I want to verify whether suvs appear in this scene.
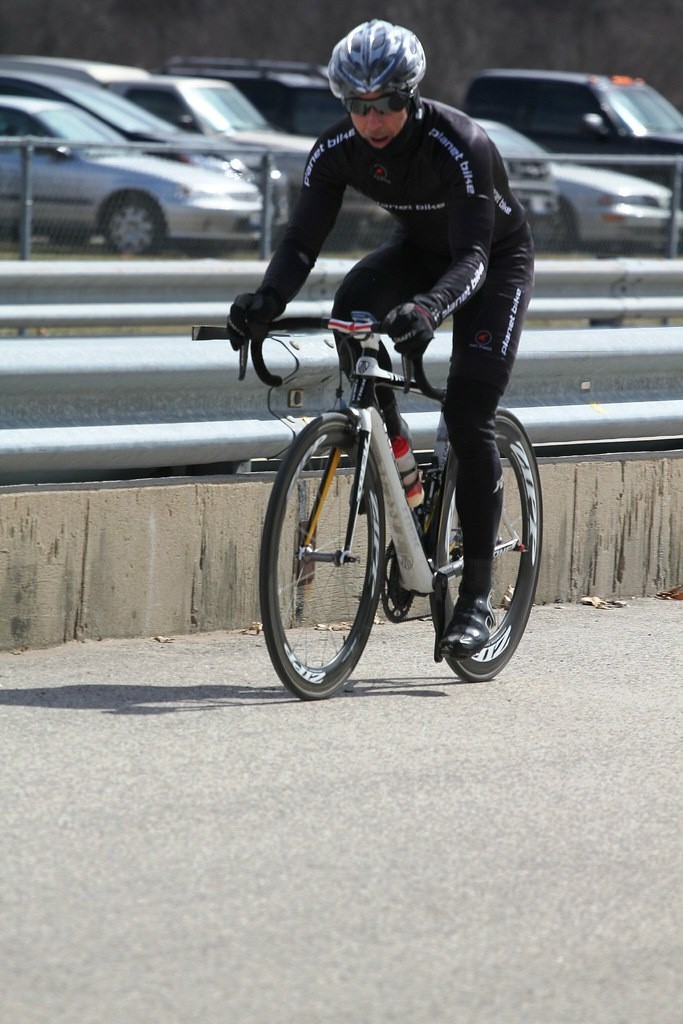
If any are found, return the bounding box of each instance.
[168,59,558,244]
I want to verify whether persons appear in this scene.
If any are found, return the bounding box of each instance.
[226,20,535,657]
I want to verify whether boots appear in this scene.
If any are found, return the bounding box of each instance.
[349,399,413,514]
[440,555,496,660]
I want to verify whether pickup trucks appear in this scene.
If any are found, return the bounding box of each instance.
[0,51,381,244]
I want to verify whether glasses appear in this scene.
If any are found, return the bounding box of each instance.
[345,93,407,116]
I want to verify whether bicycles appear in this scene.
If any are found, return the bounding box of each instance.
[192,312,544,699]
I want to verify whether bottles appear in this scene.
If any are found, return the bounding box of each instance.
[391,437,424,507]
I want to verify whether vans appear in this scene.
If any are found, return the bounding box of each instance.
[464,68,682,196]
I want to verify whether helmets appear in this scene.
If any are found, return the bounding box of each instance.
[328,19,426,99]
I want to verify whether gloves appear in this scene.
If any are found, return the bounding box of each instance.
[382,301,433,359]
[227,287,286,351]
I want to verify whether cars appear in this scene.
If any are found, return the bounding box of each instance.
[2,65,293,231]
[478,118,683,257]
[0,94,263,256]
